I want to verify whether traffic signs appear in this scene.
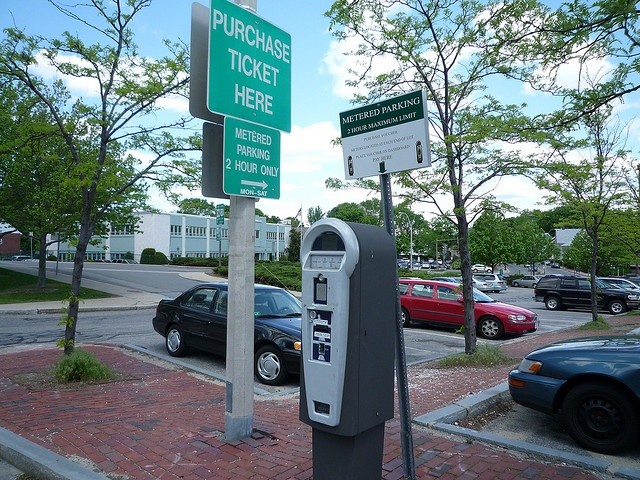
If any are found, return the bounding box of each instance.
[222,116,281,200]
[208,0,291,133]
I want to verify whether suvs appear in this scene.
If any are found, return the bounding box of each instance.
[535,275,640,314]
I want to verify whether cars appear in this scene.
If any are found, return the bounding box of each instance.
[551,262,560,268]
[398,278,539,338]
[545,260,551,266]
[512,275,543,289]
[454,277,490,290]
[529,265,537,271]
[510,326,640,455]
[470,263,493,273]
[396,257,452,271]
[430,277,457,283]
[524,264,533,267]
[597,278,640,292]
[151,282,302,385]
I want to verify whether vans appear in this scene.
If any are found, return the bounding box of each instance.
[471,273,508,293]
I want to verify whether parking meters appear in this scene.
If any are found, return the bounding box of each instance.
[297,217,395,480]
